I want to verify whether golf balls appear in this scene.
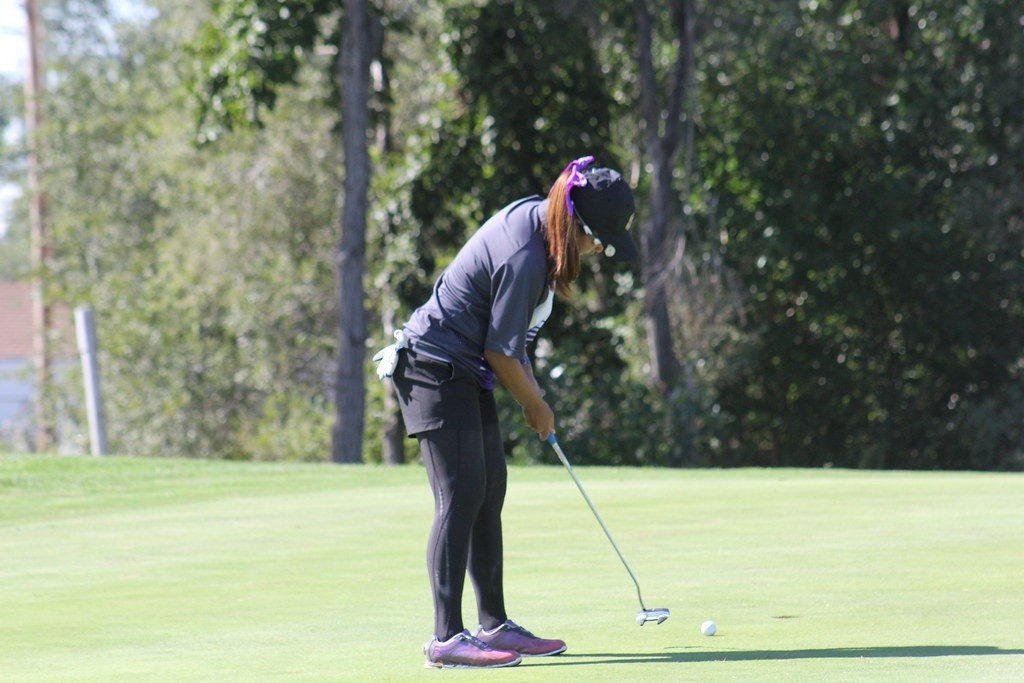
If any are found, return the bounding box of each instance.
[700,621,716,636]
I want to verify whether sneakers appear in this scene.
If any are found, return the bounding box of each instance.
[425,629,522,669]
[471,619,567,656]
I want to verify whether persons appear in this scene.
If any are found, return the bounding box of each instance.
[393,154,634,666]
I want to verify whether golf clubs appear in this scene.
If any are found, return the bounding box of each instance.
[545,432,670,626]
